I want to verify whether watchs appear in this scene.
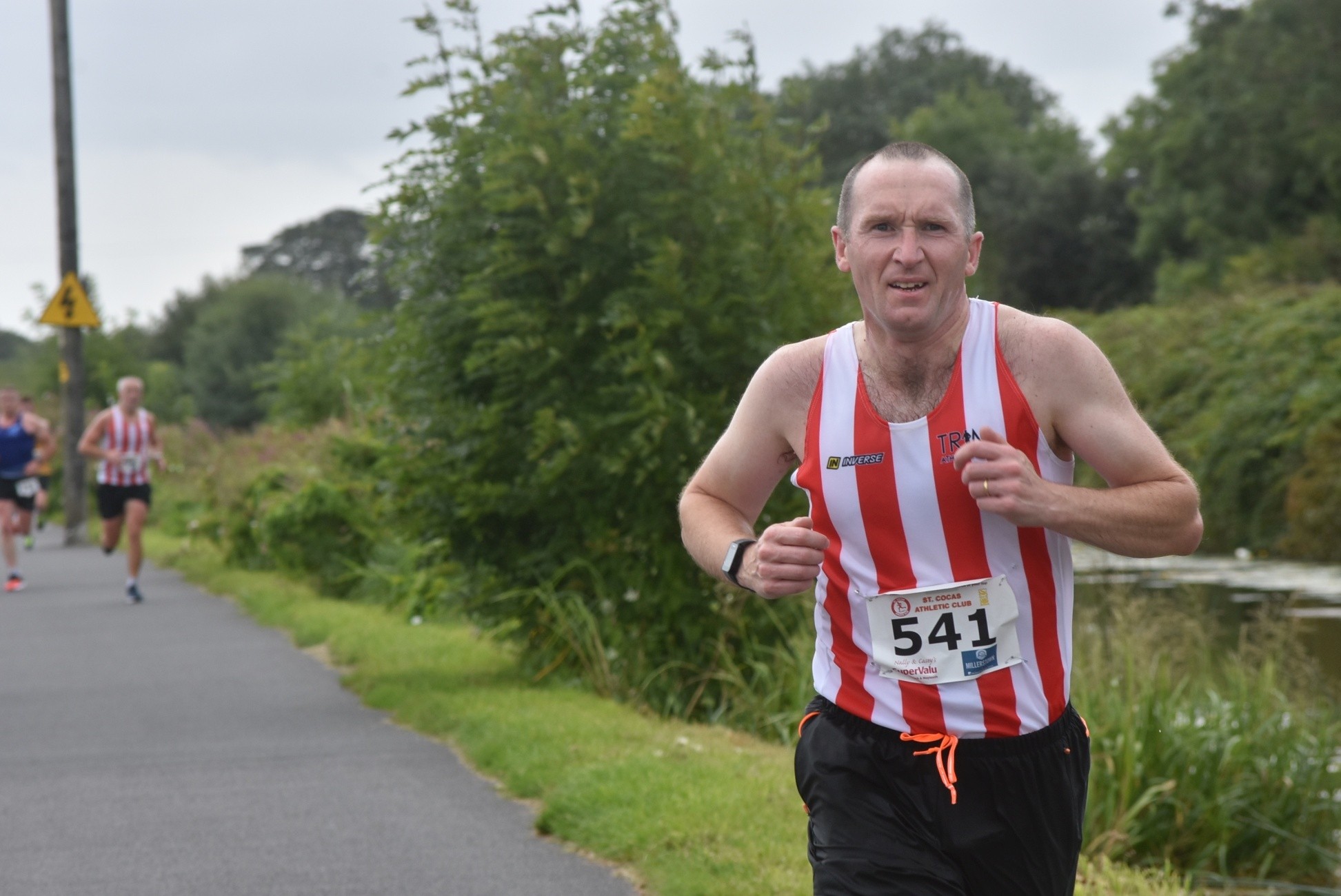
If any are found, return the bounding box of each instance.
[721,538,757,593]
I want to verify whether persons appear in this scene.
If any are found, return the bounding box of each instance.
[0,389,58,590]
[76,376,168,604]
[678,141,1204,896]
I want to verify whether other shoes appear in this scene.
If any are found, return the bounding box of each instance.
[4,574,26,592]
[102,549,114,555]
[22,534,33,551]
[123,586,142,605]
[34,510,47,530]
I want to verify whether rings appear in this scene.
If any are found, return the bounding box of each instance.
[983,479,991,498]
